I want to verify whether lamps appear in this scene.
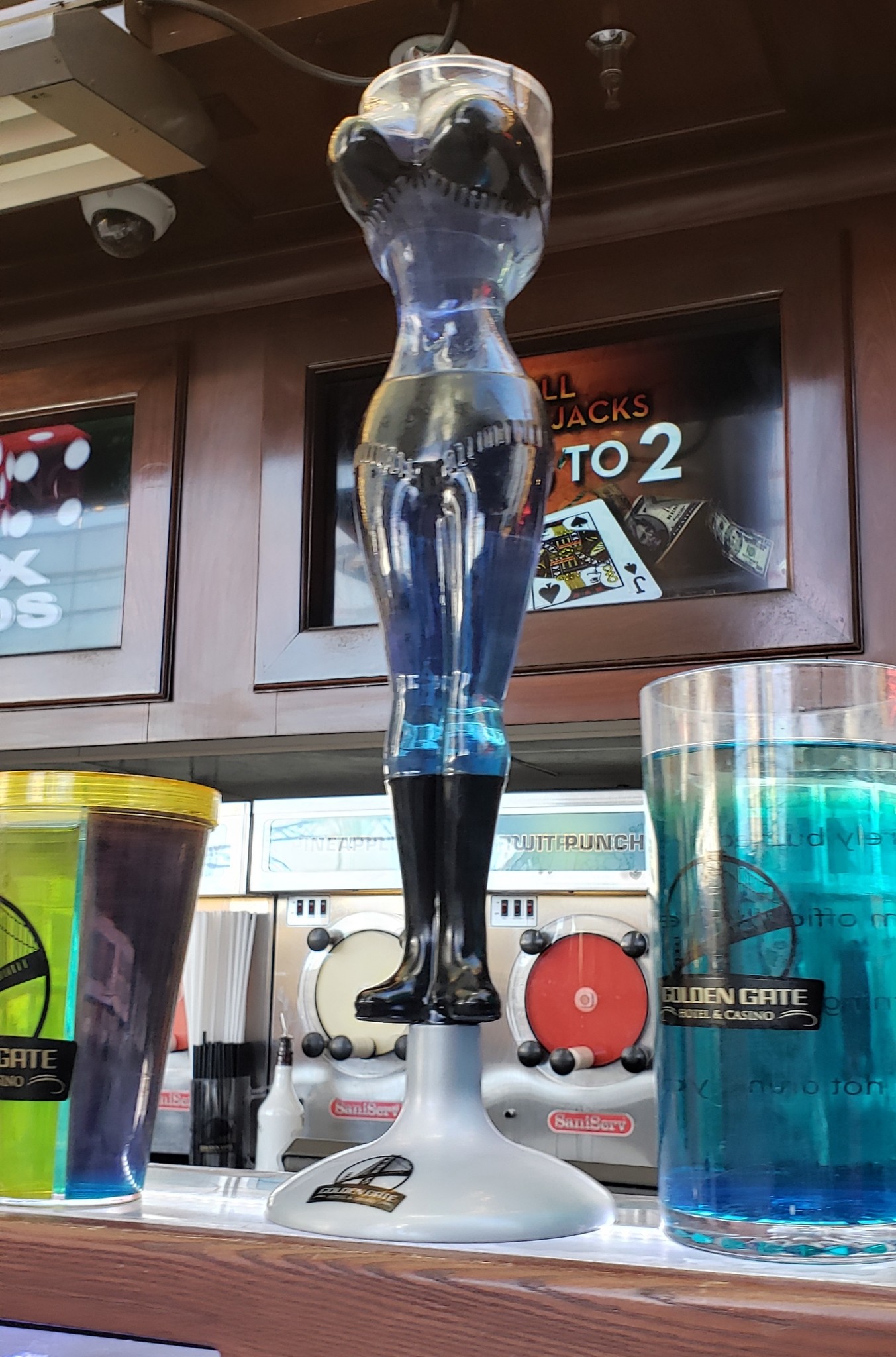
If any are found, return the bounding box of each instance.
[0,5,213,217]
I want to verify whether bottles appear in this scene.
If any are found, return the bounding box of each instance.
[255,1012,305,1172]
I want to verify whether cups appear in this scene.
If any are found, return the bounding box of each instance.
[0,770,221,1207]
[636,659,896,1266]
[187,1076,250,1168]
